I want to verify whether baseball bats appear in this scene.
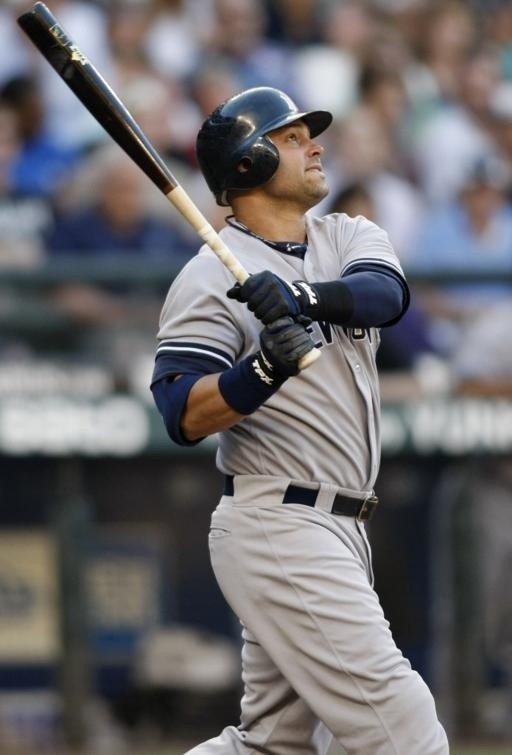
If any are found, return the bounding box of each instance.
[17,0,321,370]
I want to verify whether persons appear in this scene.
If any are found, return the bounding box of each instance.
[149,85,450,754]
[0,0,512,403]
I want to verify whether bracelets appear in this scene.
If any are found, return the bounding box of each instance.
[217,348,291,417]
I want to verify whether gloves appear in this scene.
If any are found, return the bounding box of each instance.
[227,268,323,325]
[239,317,311,390]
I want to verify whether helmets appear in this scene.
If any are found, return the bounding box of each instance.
[196,88,332,205]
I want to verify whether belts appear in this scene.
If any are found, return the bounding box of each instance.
[221,477,378,520]
